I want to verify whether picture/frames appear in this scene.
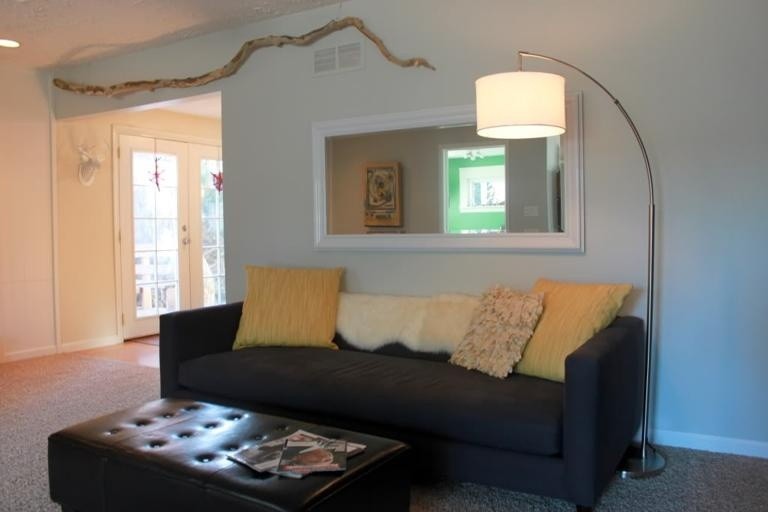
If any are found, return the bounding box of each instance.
[360,162,404,227]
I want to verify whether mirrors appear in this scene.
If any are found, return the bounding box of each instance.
[311,91,585,256]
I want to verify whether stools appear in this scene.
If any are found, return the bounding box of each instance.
[48,396,413,512]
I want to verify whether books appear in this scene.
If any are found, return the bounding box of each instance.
[226,429,368,480]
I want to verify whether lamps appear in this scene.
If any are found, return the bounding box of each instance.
[474,51,665,479]
[77,143,101,186]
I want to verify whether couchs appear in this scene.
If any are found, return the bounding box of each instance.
[160,264,646,512]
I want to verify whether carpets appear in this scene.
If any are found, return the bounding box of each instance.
[1,351,768,511]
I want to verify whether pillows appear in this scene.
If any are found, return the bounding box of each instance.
[336,291,481,355]
[515,278,632,384]
[233,264,345,351]
[448,284,544,380]
